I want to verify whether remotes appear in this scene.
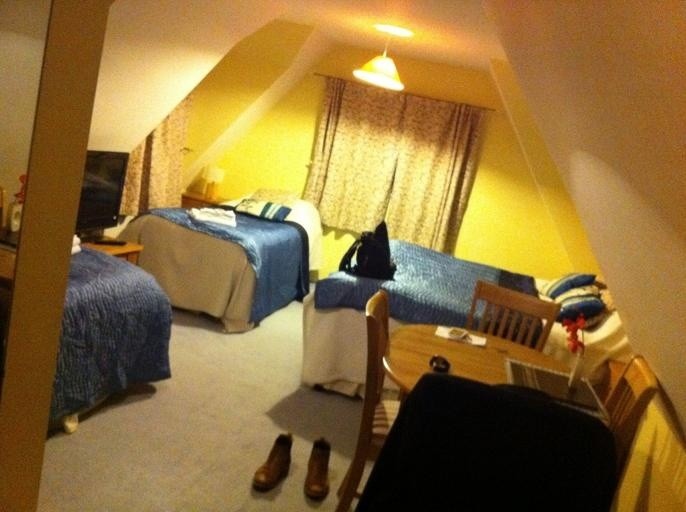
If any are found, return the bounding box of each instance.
[94,240,126,245]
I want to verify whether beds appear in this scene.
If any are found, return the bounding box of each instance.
[301,237,632,391]
[0,231,172,434]
[130,204,323,333]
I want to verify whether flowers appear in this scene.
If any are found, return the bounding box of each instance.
[562,313,586,355]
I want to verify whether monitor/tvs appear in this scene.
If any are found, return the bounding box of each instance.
[74,150,129,243]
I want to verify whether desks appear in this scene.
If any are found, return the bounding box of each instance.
[382,323,613,430]
[78,235,145,267]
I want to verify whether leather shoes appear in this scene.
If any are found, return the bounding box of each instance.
[252,434,292,492]
[303,439,330,501]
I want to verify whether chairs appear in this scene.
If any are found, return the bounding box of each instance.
[602,352,660,460]
[351,373,618,511]
[465,279,562,352]
[334,289,415,512]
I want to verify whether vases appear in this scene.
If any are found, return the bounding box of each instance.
[568,354,587,389]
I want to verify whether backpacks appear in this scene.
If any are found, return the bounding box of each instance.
[339,219,397,281]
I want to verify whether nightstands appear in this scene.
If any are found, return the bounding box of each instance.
[181,191,228,208]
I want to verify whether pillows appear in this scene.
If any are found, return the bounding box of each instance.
[537,271,606,330]
[235,199,290,221]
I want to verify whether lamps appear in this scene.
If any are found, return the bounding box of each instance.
[352,38,405,93]
